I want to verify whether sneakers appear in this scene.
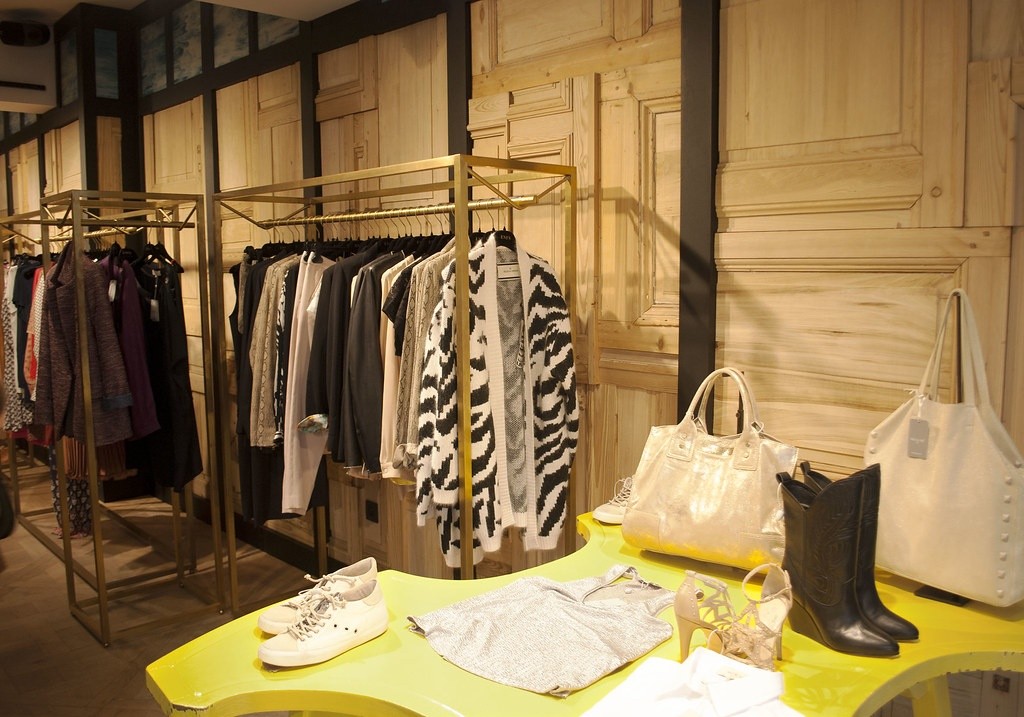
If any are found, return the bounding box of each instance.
[594,475,636,525]
[259,579,387,667]
[258,557,377,635]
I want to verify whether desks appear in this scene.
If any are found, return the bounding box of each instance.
[136,503,1024,717]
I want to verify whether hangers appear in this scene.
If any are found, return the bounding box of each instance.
[244,198,517,255]
[3,229,185,274]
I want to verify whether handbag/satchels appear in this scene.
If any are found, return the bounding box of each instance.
[864,288,1024,608]
[621,366,799,574]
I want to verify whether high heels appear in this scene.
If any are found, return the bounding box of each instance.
[727,562,793,666]
[674,570,750,663]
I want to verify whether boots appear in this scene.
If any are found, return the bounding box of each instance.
[800,461,918,643]
[776,472,900,657]
